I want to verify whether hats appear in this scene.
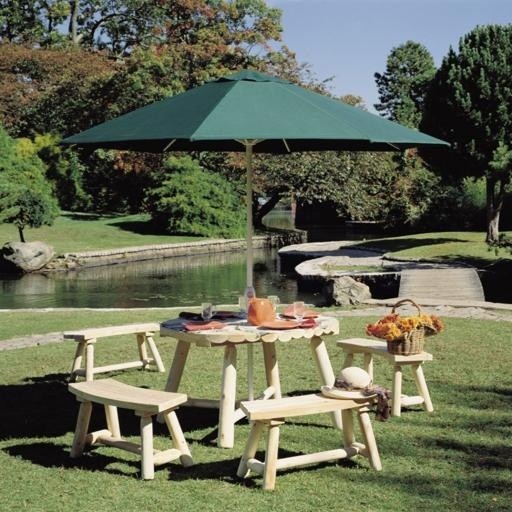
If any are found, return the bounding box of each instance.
[320,366,379,399]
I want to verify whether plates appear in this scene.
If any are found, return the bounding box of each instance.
[261,320,301,329]
[283,311,321,318]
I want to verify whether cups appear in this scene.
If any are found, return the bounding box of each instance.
[238,295,248,313]
[201,302,217,321]
[292,300,305,321]
[267,295,280,312]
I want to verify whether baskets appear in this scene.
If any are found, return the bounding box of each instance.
[386,298,424,355]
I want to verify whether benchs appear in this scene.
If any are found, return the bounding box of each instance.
[337,336,436,416]
[237,390,383,490]
[67,378,193,481]
[63,321,165,381]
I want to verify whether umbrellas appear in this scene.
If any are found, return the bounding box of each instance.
[54,66,455,401]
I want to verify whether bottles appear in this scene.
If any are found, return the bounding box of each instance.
[246,286,254,303]
